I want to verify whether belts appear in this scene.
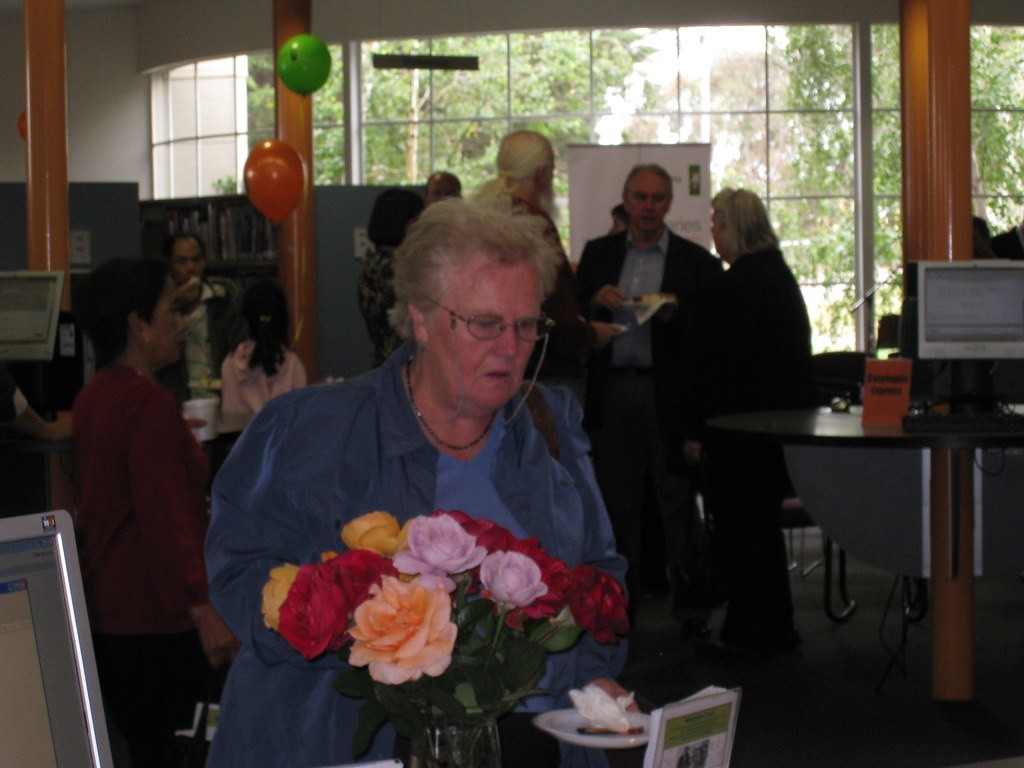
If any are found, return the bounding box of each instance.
[608,365,652,377]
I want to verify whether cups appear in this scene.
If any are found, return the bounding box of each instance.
[183,398,220,442]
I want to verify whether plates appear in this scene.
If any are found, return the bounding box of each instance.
[534,709,652,749]
[622,299,673,310]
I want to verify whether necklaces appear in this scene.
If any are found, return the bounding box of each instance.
[406,357,498,450]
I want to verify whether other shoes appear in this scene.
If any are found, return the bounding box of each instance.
[696,630,801,664]
[682,618,712,640]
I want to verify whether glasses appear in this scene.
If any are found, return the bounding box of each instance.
[421,293,556,341]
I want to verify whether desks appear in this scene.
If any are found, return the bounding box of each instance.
[708,403,1024,701]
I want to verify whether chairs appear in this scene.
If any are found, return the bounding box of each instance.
[772,352,873,623]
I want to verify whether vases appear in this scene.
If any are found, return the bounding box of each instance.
[405,715,500,768]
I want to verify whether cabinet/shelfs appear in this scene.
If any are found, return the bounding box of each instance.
[151,185,428,391]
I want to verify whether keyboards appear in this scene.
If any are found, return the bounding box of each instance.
[902,411,1024,435]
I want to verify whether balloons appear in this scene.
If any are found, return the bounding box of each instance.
[244,141,304,223]
[277,34,331,95]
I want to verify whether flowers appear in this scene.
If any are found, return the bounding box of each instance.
[255,506,628,724]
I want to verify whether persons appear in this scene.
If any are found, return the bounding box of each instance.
[71,256,239,768]
[573,165,722,634]
[0,367,75,511]
[155,233,249,390]
[682,190,812,667]
[973,215,1024,260]
[203,197,639,768]
[472,130,624,382]
[359,171,463,373]
[208,282,308,499]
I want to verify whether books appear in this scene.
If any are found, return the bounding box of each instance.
[169,204,274,260]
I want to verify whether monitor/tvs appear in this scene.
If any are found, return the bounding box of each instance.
[1,269,64,362]
[0,510,116,768]
[902,261,1024,411]
